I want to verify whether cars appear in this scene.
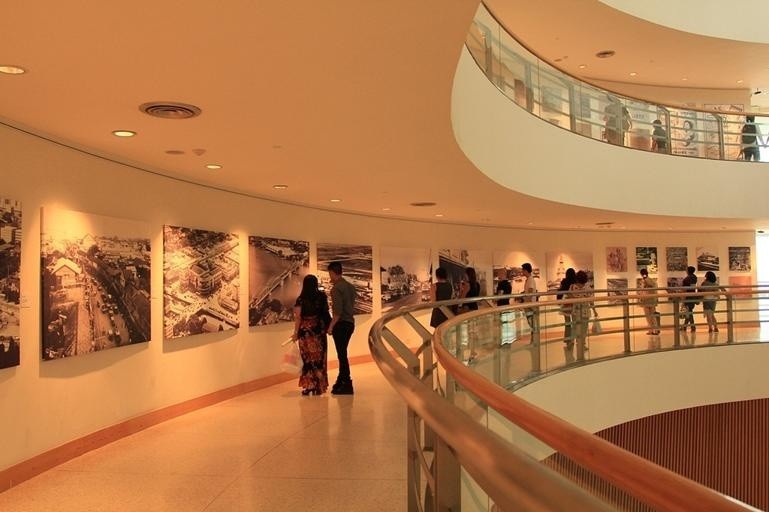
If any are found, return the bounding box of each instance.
[382,282,431,302]
[91,277,120,342]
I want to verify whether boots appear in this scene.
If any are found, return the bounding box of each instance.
[330,377,354,395]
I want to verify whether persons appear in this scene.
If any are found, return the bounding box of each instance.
[697,271,728,333]
[603,92,633,146]
[679,266,699,330]
[638,269,660,335]
[427,261,598,353]
[288,274,332,397]
[739,115,768,161]
[652,119,668,153]
[327,262,356,395]
[682,120,695,147]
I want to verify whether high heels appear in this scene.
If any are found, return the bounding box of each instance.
[302,388,320,394]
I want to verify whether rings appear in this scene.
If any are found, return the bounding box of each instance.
[329,331,332,334]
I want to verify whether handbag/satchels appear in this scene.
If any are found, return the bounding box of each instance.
[559,292,572,316]
